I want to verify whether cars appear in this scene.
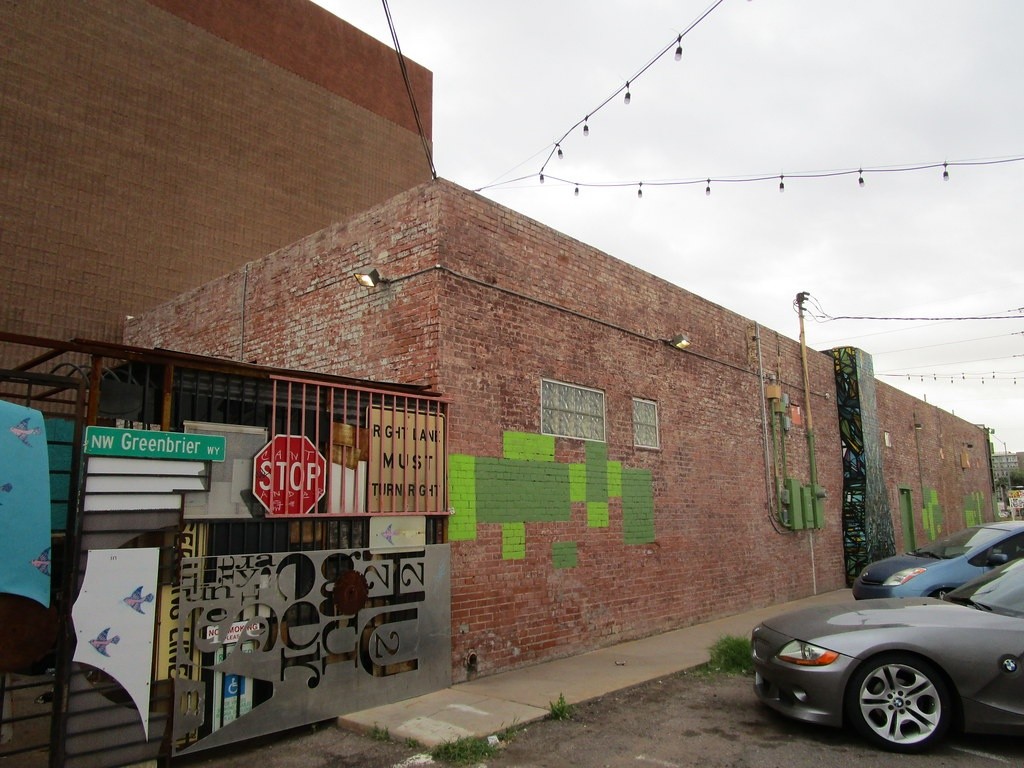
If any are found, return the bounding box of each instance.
[748,555,1024,752]
[852,518,1024,603]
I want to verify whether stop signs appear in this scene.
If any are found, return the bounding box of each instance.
[249,437,325,516]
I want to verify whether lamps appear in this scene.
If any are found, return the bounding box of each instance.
[667,333,691,349]
[963,442,973,448]
[354,267,388,288]
[913,424,922,429]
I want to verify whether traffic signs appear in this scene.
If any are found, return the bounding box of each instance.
[86,425,226,465]
